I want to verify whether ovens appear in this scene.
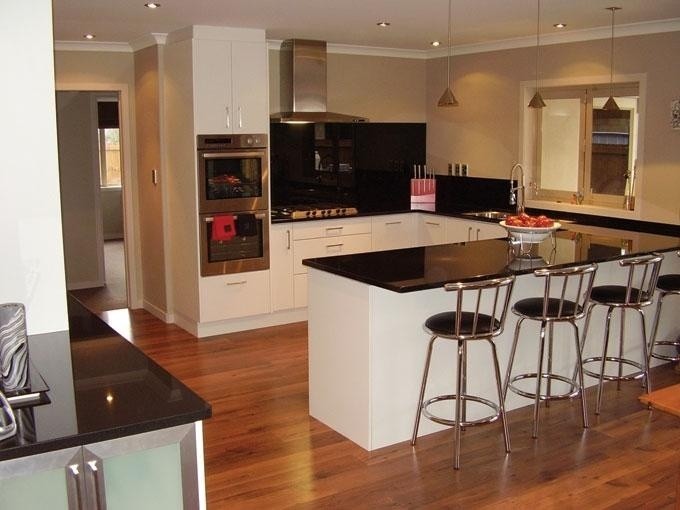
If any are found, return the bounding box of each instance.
[198,134,269,277]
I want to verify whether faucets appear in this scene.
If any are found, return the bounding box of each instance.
[509,163,524,213]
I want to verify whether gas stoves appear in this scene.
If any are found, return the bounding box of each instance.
[272,197,357,219]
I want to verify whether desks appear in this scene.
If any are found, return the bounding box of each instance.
[637,382,680,441]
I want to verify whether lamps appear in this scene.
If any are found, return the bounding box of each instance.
[528,0,547,108]
[437,0,459,108]
[602,7,621,114]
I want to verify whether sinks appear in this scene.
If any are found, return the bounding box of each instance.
[463,210,509,221]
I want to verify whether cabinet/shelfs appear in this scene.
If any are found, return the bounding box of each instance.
[269,219,294,327]
[295,216,372,324]
[169,269,272,339]
[0,408,209,510]
[165,38,270,134]
[373,211,419,252]
[447,216,508,244]
[419,213,447,247]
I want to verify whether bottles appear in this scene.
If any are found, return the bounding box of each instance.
[623,169,631,209]
[631,166,636,210]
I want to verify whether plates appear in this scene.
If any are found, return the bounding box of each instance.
[500,221,561,242]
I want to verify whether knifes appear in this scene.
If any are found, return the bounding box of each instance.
[413,164,435,178]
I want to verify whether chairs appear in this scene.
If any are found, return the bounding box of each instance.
[411,275,511,470]
[648,249,680,368]
[570,251,664,415]
[502,263,599,438]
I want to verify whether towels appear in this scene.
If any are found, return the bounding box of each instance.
[236,215,257,237]
[212,215,236,240]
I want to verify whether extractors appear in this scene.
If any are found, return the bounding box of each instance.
[270,38,369,125]
